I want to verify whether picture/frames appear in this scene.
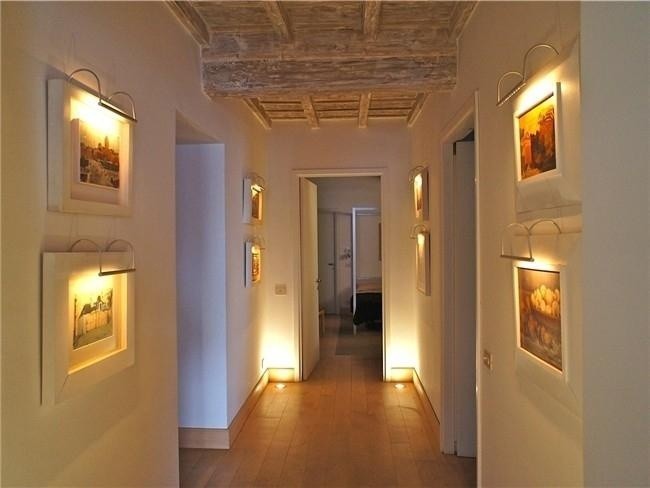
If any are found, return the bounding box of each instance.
[512,260,569,380]
[513,82,562,185]
[413,167,432,297]
[46,78,134,218]
[39,252,135,405]
[242,177,263,288]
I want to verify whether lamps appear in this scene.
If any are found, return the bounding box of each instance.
[499,217,563,264]
[69,239,138,278]
[67,68,139,125]
[494,43,561,108]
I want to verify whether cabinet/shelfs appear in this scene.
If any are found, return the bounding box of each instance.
[317,211,353,315]
